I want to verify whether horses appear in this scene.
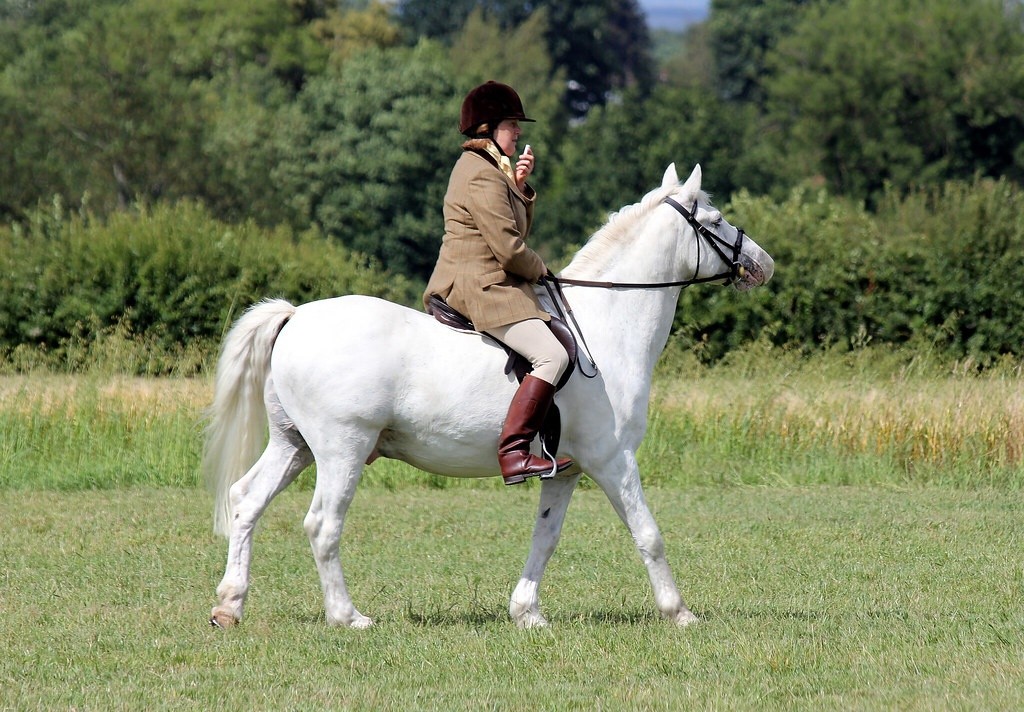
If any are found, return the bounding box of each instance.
[198,161,776,635]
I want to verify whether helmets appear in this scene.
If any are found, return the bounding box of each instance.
[460,81,536,135]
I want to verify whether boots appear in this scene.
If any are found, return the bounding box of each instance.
[497,373,574,485]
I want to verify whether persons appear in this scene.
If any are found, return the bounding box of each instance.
[423,80,573,485]
